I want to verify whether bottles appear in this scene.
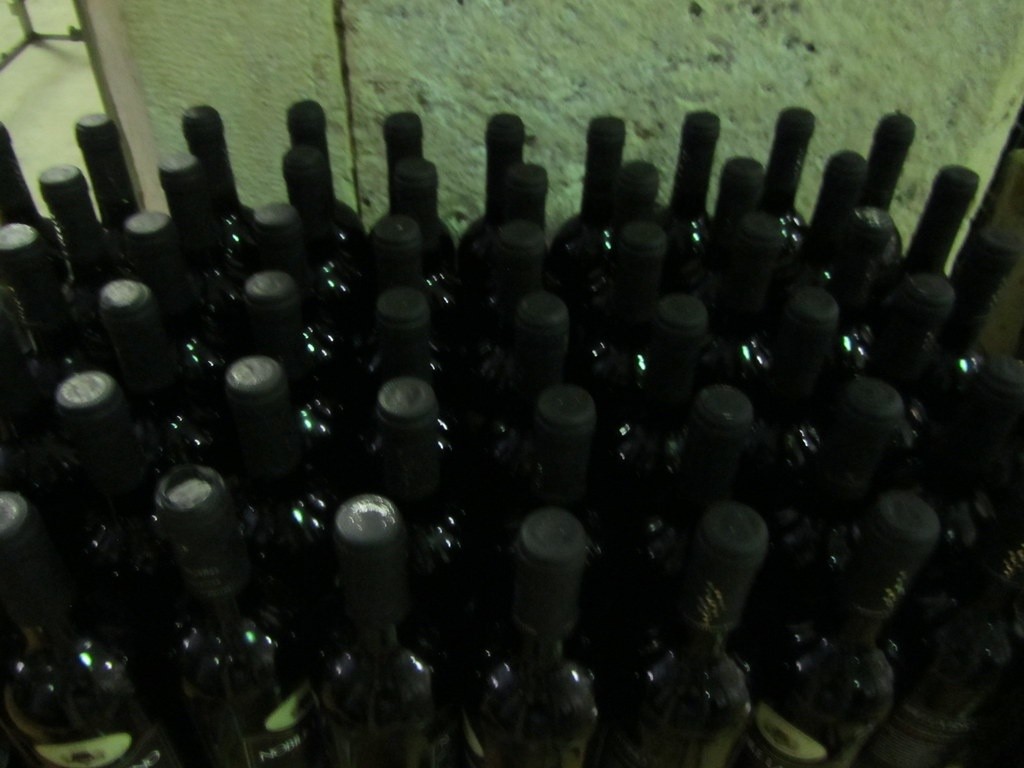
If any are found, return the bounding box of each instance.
[0,88,1024,768]
